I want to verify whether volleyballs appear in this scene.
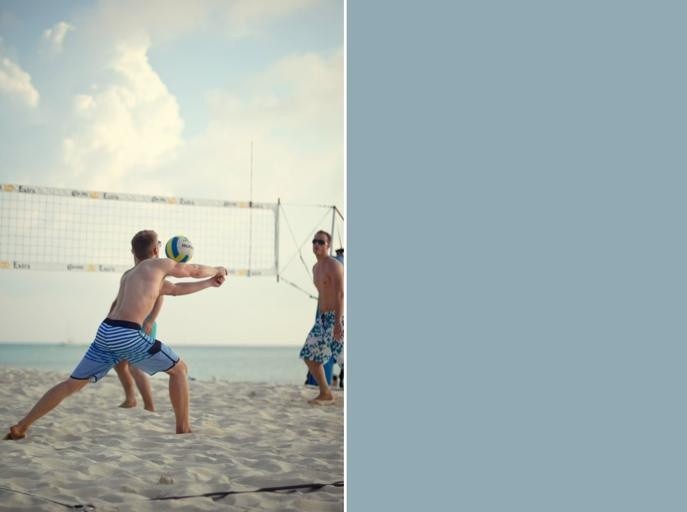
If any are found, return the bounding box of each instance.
[165,237,193,262]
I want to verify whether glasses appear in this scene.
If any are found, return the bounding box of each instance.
[312,239,328,246]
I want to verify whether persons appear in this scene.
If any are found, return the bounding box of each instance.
[10,229,228,439]
[299,230,344,405]
[109,246,163,412]
